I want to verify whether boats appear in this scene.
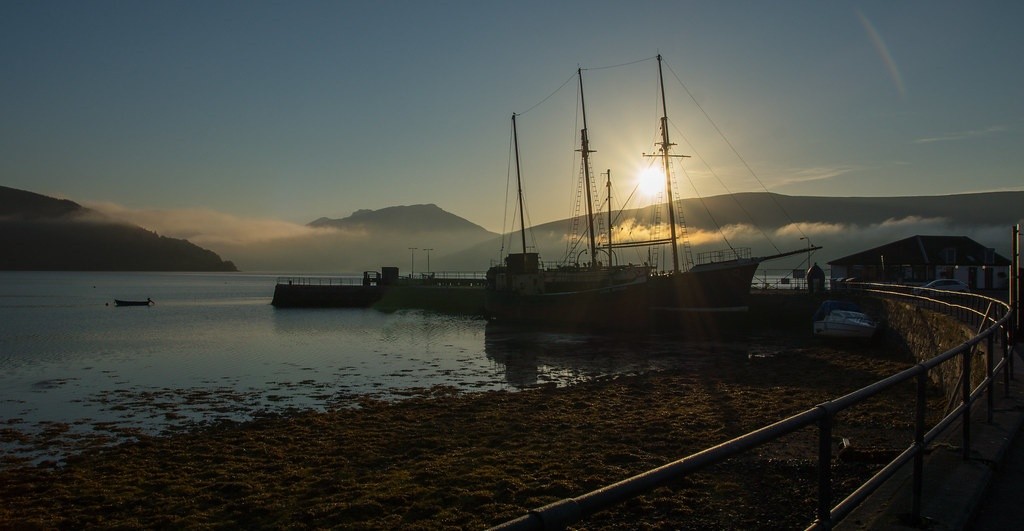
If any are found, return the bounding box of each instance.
[115,298,155,306]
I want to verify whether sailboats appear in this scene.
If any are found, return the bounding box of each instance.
[480,49,824,331]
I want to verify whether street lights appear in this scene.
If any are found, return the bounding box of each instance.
[424,248,434,278]
[800,237,811,269]
[409,247,417,279]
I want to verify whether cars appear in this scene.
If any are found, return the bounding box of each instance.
[912,278,970,297]
